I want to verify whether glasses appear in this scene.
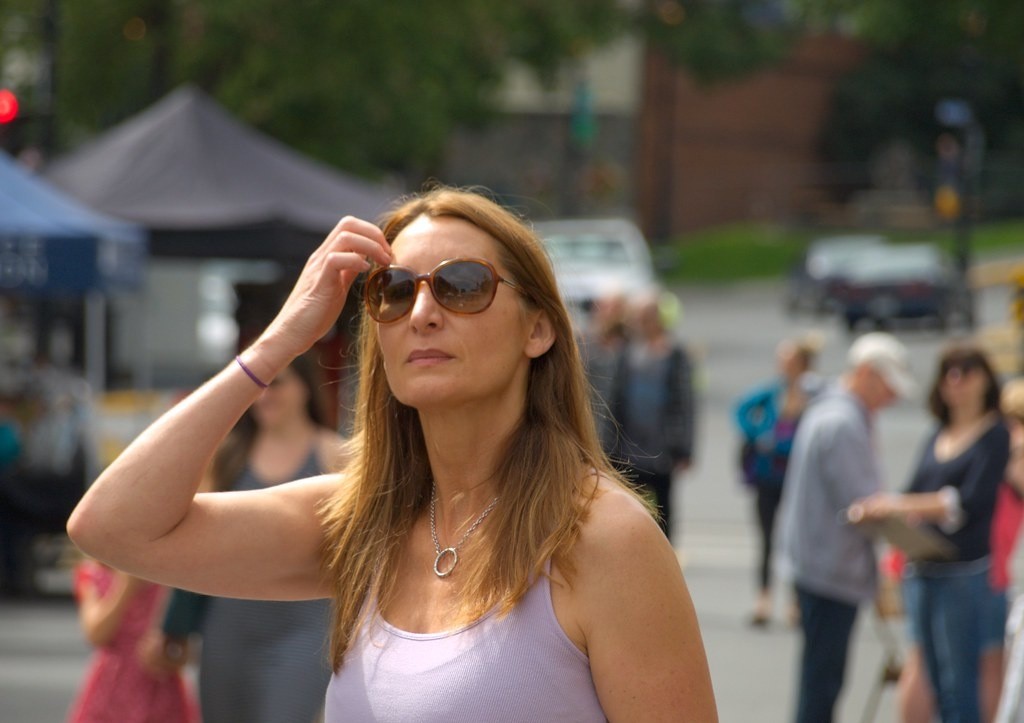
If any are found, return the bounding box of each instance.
[363,257,529,324]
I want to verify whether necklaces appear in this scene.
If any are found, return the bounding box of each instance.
[430,476,515,579]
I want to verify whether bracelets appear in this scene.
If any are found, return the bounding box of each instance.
[235,354,270,395]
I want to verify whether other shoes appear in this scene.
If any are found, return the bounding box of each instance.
[786,603,800,627]
[752,601,773,626]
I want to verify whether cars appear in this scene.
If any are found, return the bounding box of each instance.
[786,226,956,331]
[516,215,654,325]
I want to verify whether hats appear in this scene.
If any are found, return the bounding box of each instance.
[848,333,919,400]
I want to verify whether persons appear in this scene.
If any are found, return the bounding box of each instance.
[65,186,718,722]
[80,293,694,720]
[734,328,1024,722]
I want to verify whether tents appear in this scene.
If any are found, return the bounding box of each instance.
[0,81,409,610]
